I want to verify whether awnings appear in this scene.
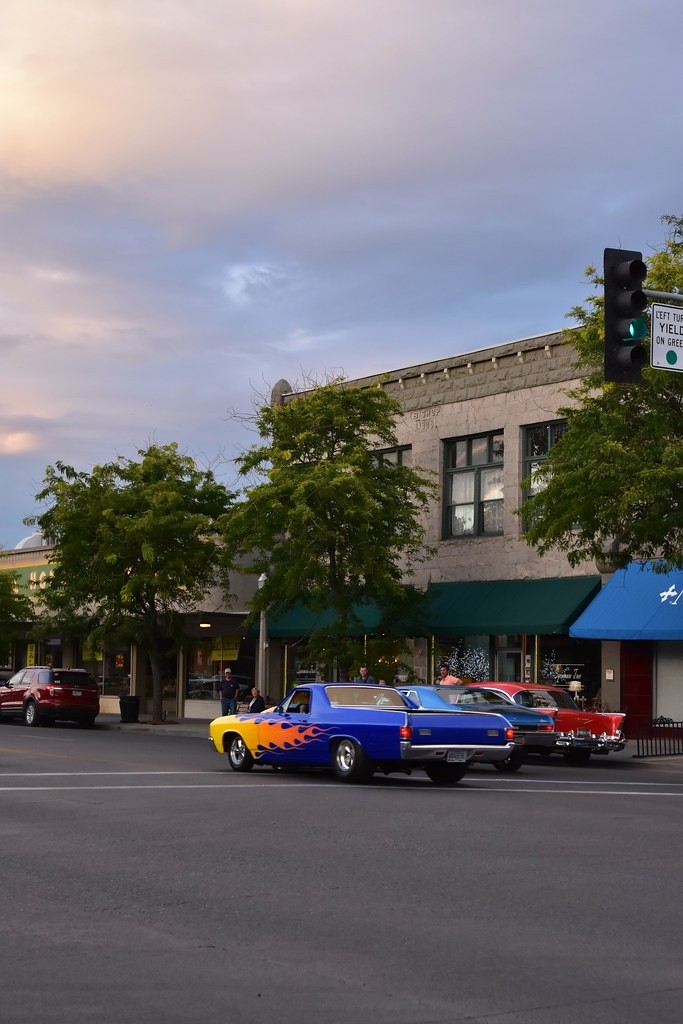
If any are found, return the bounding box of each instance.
[245,561,683,642]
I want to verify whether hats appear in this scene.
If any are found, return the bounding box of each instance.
[224,667,231,673]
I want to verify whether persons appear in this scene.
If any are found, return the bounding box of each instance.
[216,668,240,716]
[438,664,463,704]
[354,666,386,686]
[246,687,266,713]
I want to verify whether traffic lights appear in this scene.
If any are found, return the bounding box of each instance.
[603,246,650,386]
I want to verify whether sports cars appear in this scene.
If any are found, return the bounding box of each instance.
[463,680,628,768]
[209,683,514,789]
[395,685,558,772]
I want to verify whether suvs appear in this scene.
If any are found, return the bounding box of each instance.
[0,665,101,730]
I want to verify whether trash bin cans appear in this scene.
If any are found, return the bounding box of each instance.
[118,695,141,723]
